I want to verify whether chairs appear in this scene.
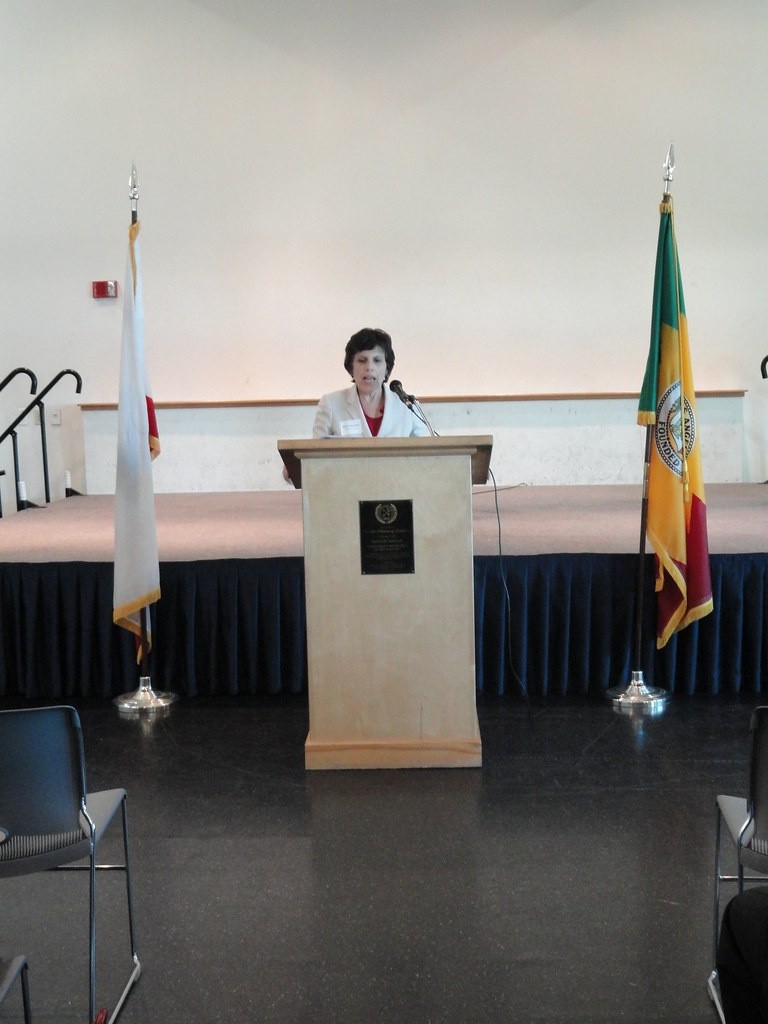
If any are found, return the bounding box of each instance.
[0,705,143,1024]
[704,704,768,1024]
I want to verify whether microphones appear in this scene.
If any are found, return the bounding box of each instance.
[389,380,412,410]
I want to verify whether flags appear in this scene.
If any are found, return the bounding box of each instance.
[635,195,714,650]
[112,221,163,662]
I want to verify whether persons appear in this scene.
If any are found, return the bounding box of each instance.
[282,327,432,483]
